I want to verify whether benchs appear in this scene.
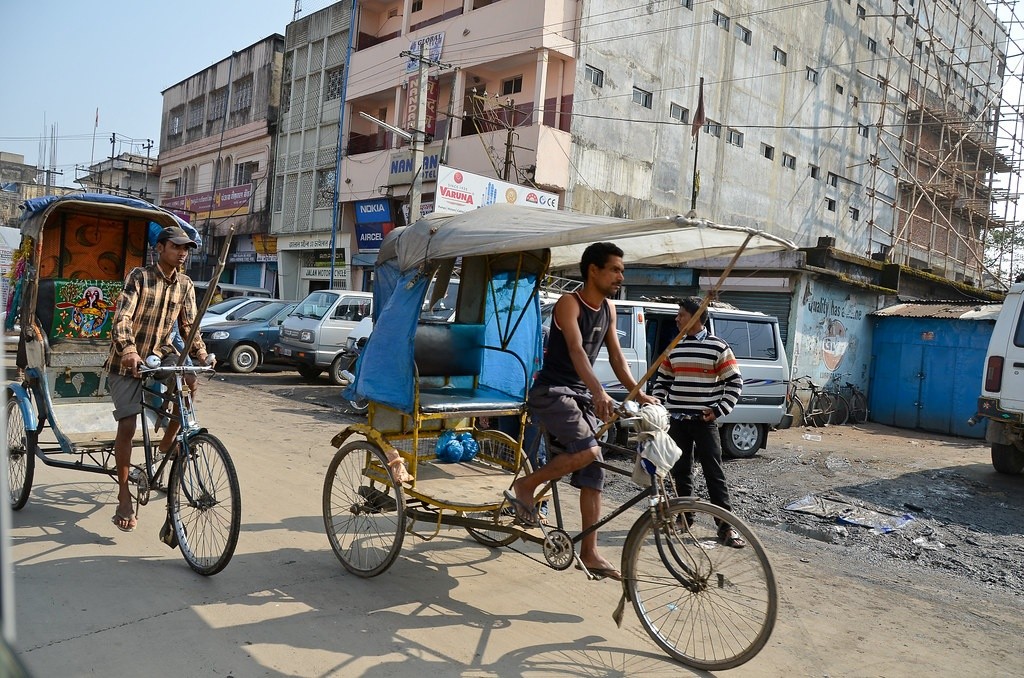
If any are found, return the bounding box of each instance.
[414,322,529,417]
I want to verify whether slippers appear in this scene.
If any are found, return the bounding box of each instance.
[157,448,197,460]
[116,505,137,531]
[503,490,538,526]
[661,520,689,535]
[718,528,746,547]
[575,559,622,581]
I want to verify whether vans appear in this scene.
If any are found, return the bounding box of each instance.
[192,279,272,311]
[348,275,563,337]
[540,296,795,460]
[274,288,430,386]
[969,273,1024,477]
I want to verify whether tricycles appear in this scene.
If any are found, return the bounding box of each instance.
[1,191,242,576]
[322,205,779,672]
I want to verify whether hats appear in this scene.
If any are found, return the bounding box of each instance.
[157,226,197,249]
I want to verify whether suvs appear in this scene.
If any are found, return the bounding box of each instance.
[199,299,333,374]
[198,295,284,328]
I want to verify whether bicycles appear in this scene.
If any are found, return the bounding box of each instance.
[815,373,872,425]
[785,374,835,427]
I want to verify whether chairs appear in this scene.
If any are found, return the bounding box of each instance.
[36,279,124,344]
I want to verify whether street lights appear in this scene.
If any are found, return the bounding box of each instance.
[355,109,426,226]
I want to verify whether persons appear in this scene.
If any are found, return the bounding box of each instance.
[650,295,746,548]
[100,226,217,533]
[503,242,662,581]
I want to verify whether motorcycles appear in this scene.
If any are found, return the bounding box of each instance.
[339,336,371,415]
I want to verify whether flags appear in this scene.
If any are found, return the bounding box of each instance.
[692,88,704,135]
